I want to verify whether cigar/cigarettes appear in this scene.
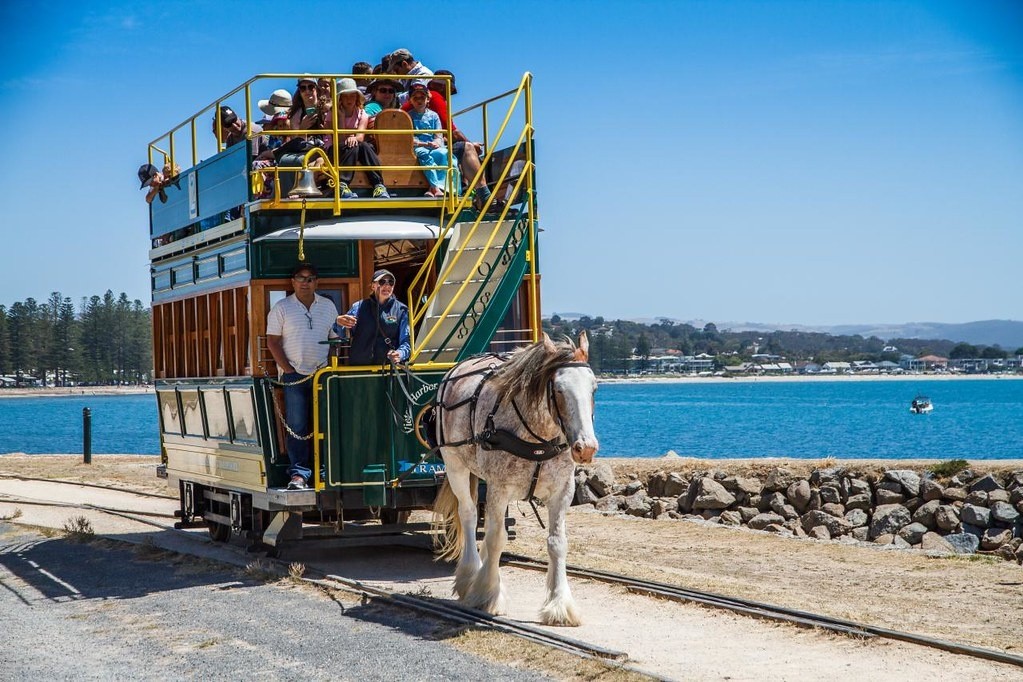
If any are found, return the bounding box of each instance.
[347,317,350,320]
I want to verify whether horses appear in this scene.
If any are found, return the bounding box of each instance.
[430,330,600,628]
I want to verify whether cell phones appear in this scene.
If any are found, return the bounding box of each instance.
[306,107,315,117]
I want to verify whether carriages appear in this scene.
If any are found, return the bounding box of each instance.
[143,71,602,629]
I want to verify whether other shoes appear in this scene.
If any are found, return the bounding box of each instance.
[424,188,445,197]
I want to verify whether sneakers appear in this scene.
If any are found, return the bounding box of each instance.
[340,182,358,198]
[475,196,506,212]
[288,476,309,489]
[373,184,390,198]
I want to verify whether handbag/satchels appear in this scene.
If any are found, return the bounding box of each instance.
[273,137,309,162]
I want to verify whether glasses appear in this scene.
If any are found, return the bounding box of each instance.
[224,118,237,127]
[378,279,395,287]
[213,129,217,133]
[299,84,316,90]
[376,87,394,93]
[295,275,315,284]
[305,312,314,330]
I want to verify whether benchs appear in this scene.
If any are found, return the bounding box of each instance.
[296,109,430,188]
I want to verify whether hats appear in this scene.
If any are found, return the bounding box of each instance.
[336,78,366,104]
[385,49,412,71]
[215,107,237,128]
[409,84,430,98]
[429,69,458,94]
[271,112,289,123]
[366,73,404,93]
[372,269,396,284]
[296,72,318,88]
[138,165,158,189]
[255,114,274,123]
[258,89,293,115]
[292,263,316,277]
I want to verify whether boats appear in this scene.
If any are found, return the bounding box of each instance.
[909,397,933,414]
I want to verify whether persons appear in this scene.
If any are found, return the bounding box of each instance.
[211,49,509,214]
[138,162,181,203]
[332,269,411,366]
[266,261,341,489]
